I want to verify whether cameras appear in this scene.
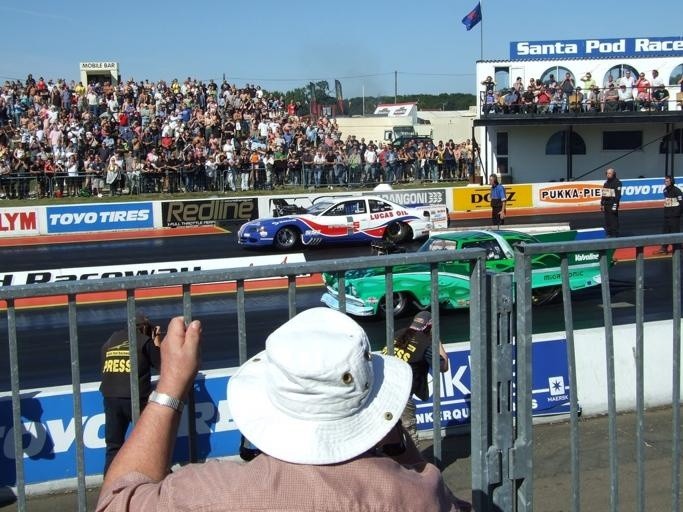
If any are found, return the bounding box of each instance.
[146,324,160,337]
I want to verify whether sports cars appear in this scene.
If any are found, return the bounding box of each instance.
[238,193,431,251]
[320,227,616,319]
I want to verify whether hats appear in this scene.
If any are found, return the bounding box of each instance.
[409,310,432,331]
[226,306,414,465]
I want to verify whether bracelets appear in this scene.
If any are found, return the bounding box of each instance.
[148,390,184,414]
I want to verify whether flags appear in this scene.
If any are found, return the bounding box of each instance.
[462,3,484,32]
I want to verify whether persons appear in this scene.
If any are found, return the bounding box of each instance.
[600,168,622,236]
[488,174,507,226]
[85,313,166,479]
[382,311,448,459]
[481,69,683,113]
[0,72,482,198]
[93,308,470,512]
[660,175,683,255]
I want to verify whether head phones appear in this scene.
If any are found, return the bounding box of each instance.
[606,168,616,176]
[668,175,675,184]
